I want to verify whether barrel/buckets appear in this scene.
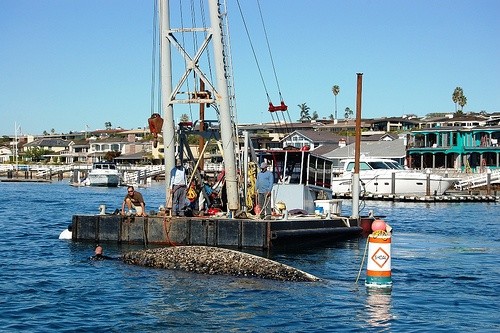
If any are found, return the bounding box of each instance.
[275,203,286,213]
[315,206,323,214]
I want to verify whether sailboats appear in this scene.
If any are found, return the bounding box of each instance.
[68,0,387,260]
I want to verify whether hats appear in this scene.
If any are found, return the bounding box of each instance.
[260,163,268,169]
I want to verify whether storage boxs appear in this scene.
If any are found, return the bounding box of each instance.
[314,199,342,217]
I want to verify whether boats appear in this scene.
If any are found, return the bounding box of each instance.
[87,162,120,187]
[330,131,463,195]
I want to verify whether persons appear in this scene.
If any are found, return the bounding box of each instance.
[187,175,217,196]
[121,185,148,217]
[168,158,187,217]
[88,243,112,259]
[255,162,274,219]
